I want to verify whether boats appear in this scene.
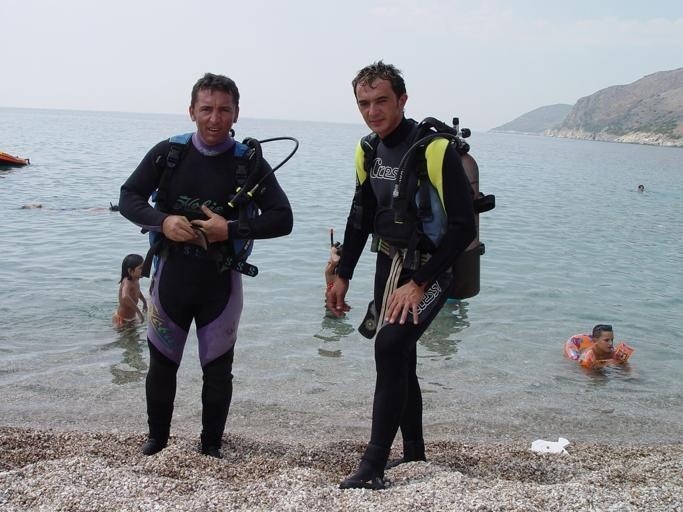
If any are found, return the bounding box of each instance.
[0,151,30,165]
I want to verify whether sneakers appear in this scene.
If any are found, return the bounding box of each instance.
[385,453,407,468]
[141,438,163,454]
[341,462,386,492]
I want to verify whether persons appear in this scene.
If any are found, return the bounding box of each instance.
[323,60,481,488]
[97,333,150,388]
[324,227,343,313]
[637,185,645,194]
[117,72,294,460]
[110,252,148,329]
[314,311,355,360]
[579,321,629,369]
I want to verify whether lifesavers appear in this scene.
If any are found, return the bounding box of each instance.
[565,335,634,369]
